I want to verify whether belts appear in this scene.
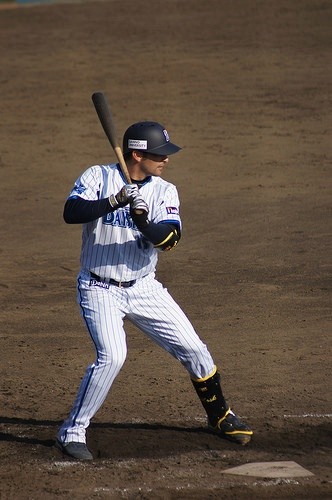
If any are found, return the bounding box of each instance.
[90,272,149,288]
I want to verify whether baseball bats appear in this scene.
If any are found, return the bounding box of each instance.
[92,90,144,216]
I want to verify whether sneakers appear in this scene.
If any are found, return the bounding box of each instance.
[208,417,253,444]
[55,438,94,461]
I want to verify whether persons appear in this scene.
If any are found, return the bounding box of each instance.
[53,120,254,462]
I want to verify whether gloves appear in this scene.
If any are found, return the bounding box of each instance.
[130,196,150,231]
[108,184,142,210]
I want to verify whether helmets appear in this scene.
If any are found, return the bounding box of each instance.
[122,121,182,155]
